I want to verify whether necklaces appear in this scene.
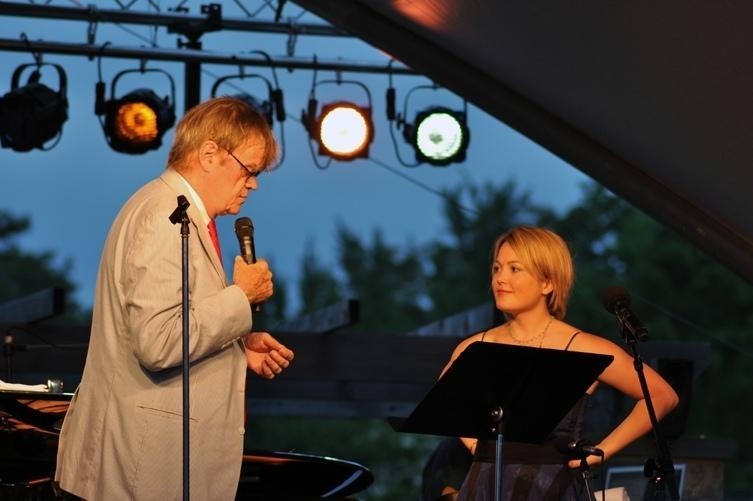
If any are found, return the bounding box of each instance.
[507,316,553,346]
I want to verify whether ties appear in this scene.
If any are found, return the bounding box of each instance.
[207,219,222,264]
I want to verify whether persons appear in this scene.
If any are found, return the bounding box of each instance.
[53,92,294,501]
[439,225,680,501]
[420,436,476,500]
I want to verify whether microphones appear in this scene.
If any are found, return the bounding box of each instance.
[234,216,263,314]
[602,284,648,344]
[556,434,604,458]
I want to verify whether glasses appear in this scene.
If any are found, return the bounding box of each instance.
[217,144,259,182]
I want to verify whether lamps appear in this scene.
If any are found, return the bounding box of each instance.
[0,40,470,169]
